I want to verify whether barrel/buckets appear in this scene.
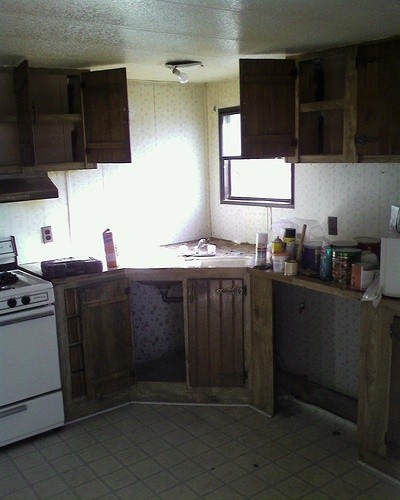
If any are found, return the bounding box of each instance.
[302,236,380,287]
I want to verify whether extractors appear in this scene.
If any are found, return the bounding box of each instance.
[0,172,59,202]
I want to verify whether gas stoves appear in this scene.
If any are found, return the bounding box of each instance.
[0,236,55,313]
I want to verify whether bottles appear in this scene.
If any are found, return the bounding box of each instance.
[254,229,295,268]
[103,229,117,269]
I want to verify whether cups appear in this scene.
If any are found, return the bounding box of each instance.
[272,252,288,272]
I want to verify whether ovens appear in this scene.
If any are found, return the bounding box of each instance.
[0,304,64,448]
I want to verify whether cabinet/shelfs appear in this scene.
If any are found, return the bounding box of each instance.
[238,34,400,163]
[63,274,277,420]
[359,303,400,482]
[1,59,132,175]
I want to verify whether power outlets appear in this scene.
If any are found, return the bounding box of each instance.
[41,226,54,244]
[328,216,338,235]
